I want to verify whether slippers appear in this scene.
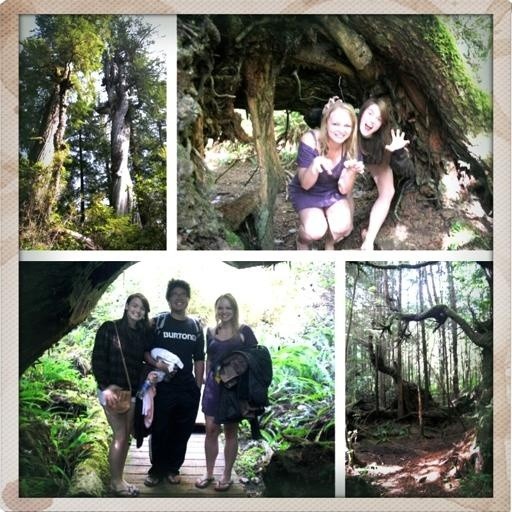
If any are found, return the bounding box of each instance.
[214,479,234,491]
[195,476,214,489]
[128,483,139,493]
[166,471,181,484]
[111,486,138,498]
[144,474,162,487]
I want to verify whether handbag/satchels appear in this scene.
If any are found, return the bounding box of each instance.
[105,390,132,415]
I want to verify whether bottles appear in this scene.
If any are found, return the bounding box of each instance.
[136,376,153,399]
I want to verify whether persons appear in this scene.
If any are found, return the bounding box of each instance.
[194,292,259,491]
[145,279,206,487]
[90,293,154,497]
[320,94,412,250]
[289,101,365,252]
